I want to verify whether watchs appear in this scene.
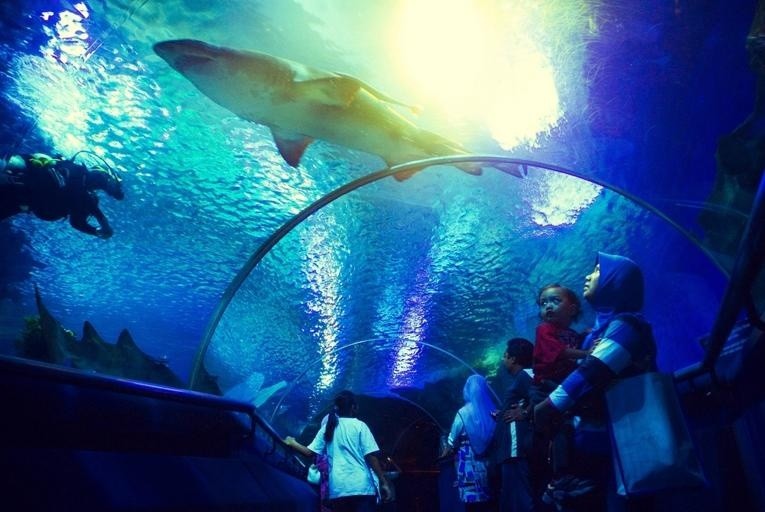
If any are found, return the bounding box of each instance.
[523,409,528,418]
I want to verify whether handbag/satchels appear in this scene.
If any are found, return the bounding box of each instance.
[604,370,707,499]
[314,456,330,503]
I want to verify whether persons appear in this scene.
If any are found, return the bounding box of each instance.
[494,338,536,511]
[534,251,659,511]
[0,153,125,238]
[534,283,605,510]
[282,390,393,512]
[440,374,497,511]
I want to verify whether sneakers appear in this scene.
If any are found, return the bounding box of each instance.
[541,473,594,510]
